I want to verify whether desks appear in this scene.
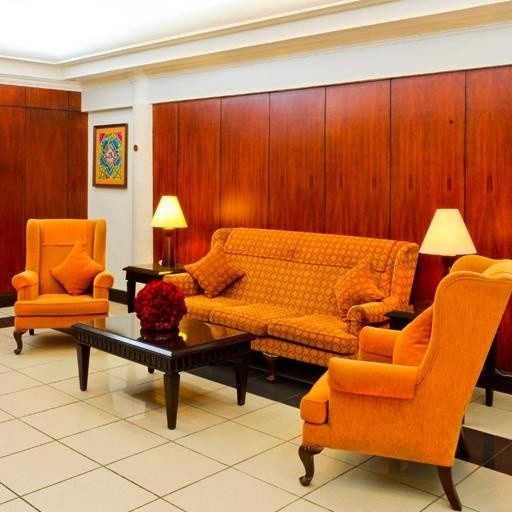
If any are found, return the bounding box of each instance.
[384,301,497,407]
[123,262,185,313]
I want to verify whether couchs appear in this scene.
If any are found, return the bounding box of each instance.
[163,227,419,383]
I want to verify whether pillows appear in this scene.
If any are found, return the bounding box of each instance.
[185,242,246,298]
[52,240,103,295]
[332,255,385,322]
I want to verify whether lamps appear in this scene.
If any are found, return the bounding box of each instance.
[420,208,477,273]
[150,195,189,267]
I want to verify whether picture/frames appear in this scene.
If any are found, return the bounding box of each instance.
[93,123,127,186]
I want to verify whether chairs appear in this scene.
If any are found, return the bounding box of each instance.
[299,255,512,511]
[11,219,114,355]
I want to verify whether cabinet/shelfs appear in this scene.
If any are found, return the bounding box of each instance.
[152,64,512,395]
[0,106,89,309]
